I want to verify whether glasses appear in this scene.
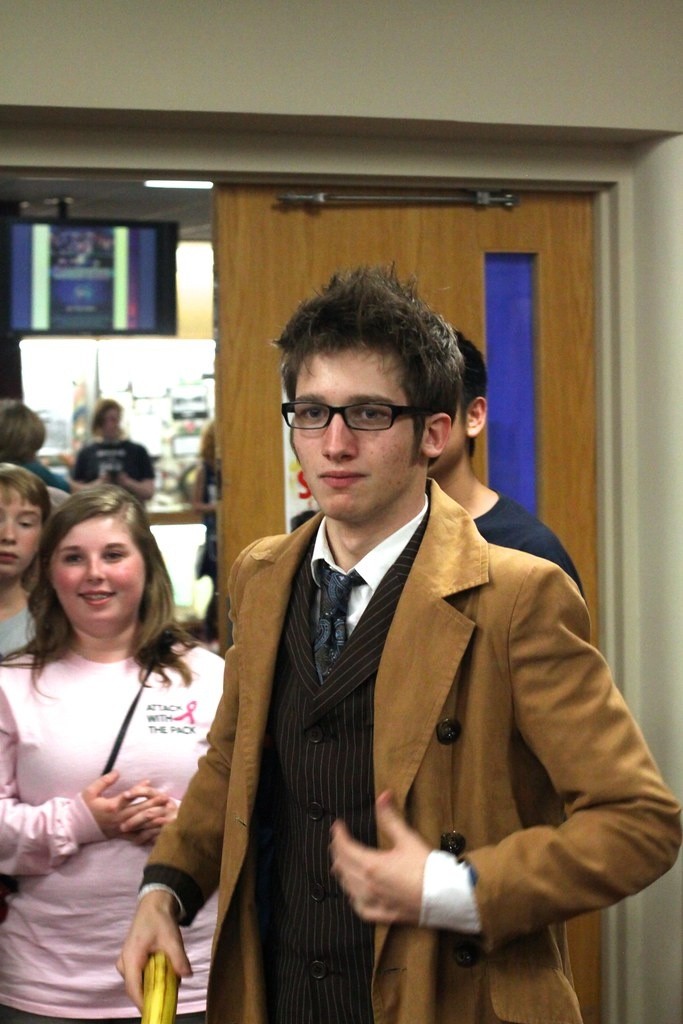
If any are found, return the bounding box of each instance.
[278,398,423,431]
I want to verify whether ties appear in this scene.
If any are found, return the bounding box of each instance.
[313,570,366,682]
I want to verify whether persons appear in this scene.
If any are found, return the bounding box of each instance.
[117,258,683,1024]
[0,393,228,1023]
[425,326,590,595]
